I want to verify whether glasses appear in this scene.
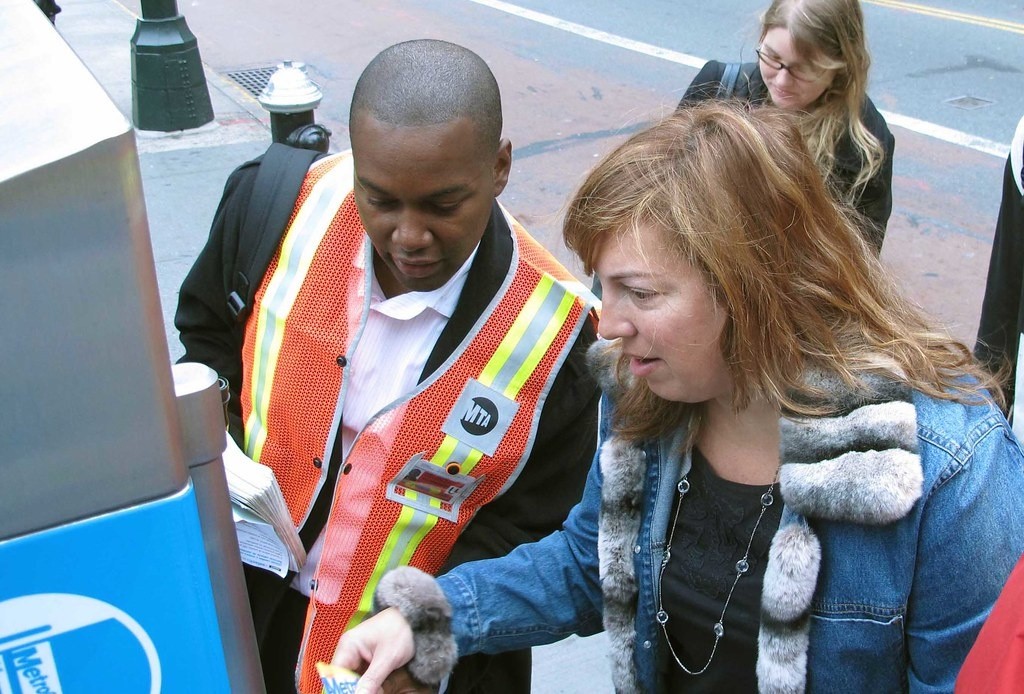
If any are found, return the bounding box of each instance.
[756,41,844,84]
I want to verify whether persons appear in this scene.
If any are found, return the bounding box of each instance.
[593,1,895,327]
[951,550,1024,694]
[968,93,1023,442]
[325,97,1024,694]
[171,33,631,694]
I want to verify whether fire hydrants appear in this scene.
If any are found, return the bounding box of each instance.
[255,59,333,156]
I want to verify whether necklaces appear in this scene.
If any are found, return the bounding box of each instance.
[657,362,805,673]
